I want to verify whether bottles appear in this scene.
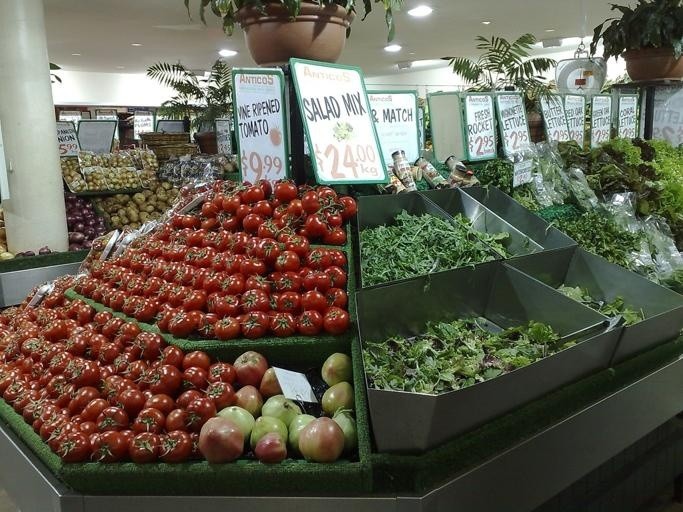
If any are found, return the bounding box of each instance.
[379,149,480,193]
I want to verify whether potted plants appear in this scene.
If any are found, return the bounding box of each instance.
[441,32,557,142]
[185,0,406,68]
[147,56,237,155]
[589,0,683,80]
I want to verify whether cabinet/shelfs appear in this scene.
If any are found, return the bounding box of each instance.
[1,152,683,512]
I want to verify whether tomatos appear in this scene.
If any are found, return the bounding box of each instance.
[0,178,357,465]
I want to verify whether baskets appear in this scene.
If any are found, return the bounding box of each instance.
[137,132,198,158]
